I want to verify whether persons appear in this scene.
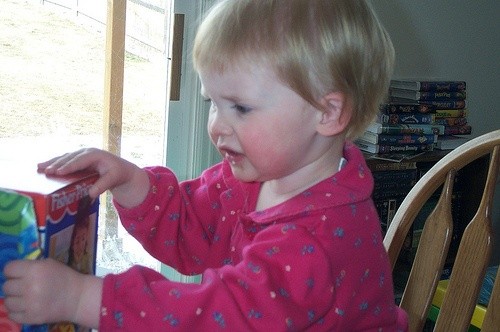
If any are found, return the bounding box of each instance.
[2,0,415,328]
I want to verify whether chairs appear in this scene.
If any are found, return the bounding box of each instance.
[382,128,500,332]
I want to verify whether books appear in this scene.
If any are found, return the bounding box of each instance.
[354,76,473,155]
